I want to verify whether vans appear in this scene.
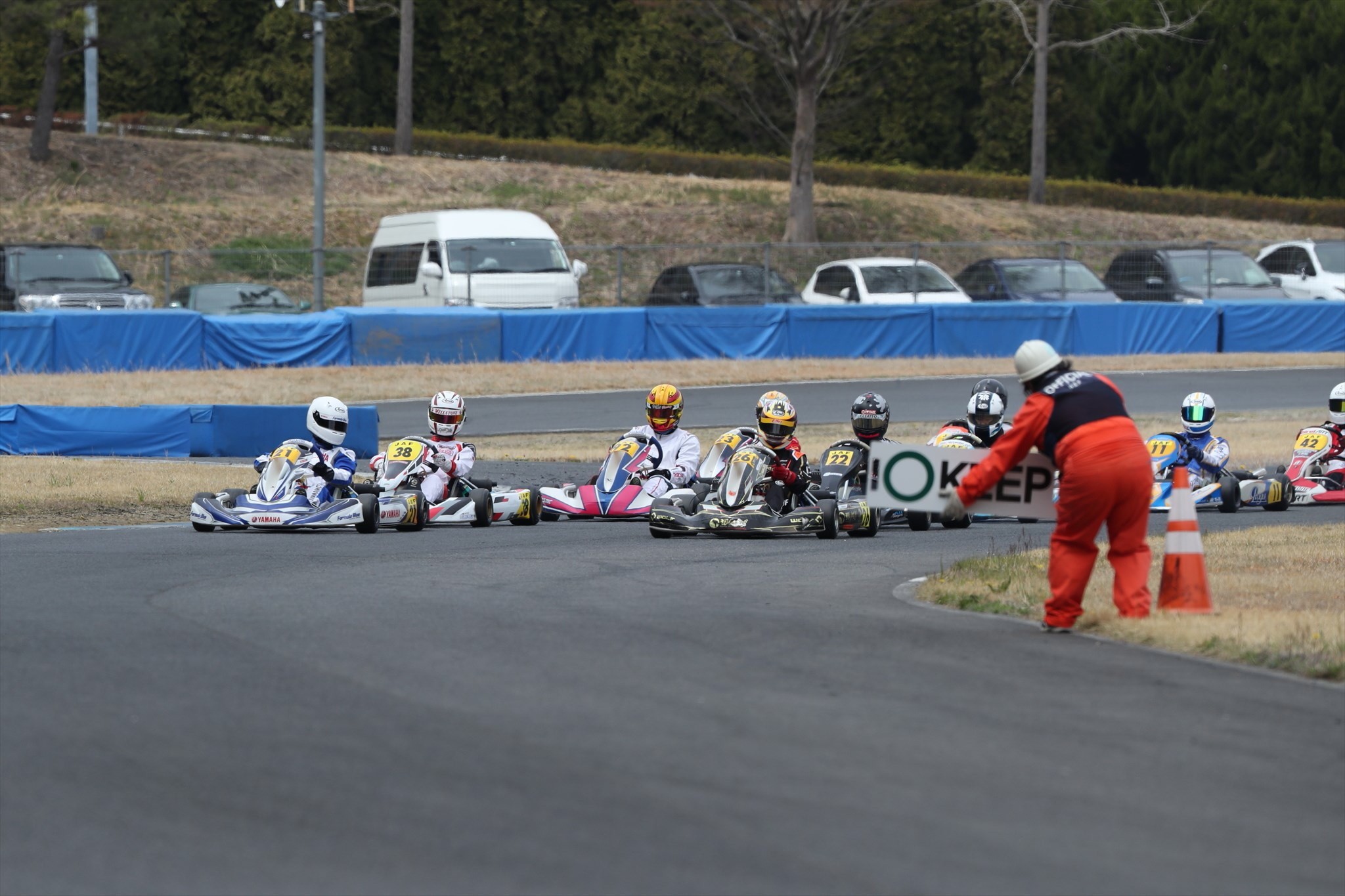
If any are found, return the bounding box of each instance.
[361,207,589,314]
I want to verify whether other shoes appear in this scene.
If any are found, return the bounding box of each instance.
[1040,620,1071,633]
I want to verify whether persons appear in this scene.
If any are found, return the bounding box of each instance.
[713,388,813,513]
[941,338,1154,635]
[809,391,905,520]
[500,240,536,272]
[1169,391,1231,493]
[928,379,1017,448]
[1276,382,1345,490]
[363,390,477,504]
[249,395,359,508]
[590,383,701,499]
[449,239,495,272]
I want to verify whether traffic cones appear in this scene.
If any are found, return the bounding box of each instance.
[1151,465,1224,617]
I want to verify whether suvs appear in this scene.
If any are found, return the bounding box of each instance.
[0,241,154,312]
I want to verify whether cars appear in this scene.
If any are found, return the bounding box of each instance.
[1247,238,1345,302]
[802,256,976,307]
[956,256,1122,303]
[646,259,808,307]
[165,282,311,314]
[1101,245,1290,302]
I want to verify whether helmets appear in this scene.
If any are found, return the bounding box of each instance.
[850,392,890,442]
[1014,339,1063,383]
[1329,382,1345,425]
[427,391,467,437]
[971,379,1007,411]
[646,384,684,434]
[967,391,1005,442]
[757,400,797,449]
[307,396,348,445]
[755,391,790,426]
[1181,392,1216,434]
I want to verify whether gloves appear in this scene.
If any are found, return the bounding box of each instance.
[432,453,452,473]
[257,461,267,473]
[1336,435,1345,449]
[312,462,334,482]
[374,459,385,470]
[771,464,796,486]
[938,487,968,521]
[1186,446,1203,463]
[647,469,671,481]
